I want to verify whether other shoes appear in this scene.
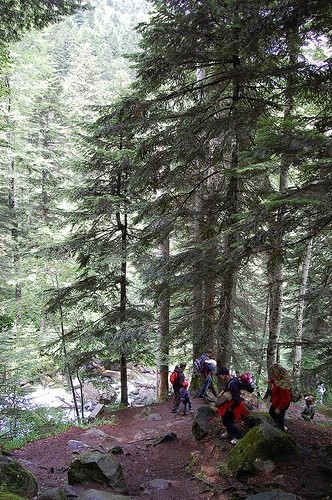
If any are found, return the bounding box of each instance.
[198,394,206,398]
[274,421,288,430]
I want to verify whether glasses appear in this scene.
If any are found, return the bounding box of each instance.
[269,372,274,377]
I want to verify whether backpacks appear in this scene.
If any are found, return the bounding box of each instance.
[193,354,207,371]
[238,371,256,393]
[170,369,178,386]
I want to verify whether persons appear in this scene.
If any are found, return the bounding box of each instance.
[179,381,193,416]
[318,383,326,405]
[268,361,292,433]
[215,367,250,445]
[204,355,218,381]
[199,348,213,398]
[299,395,315,423]
[169,361,187,413]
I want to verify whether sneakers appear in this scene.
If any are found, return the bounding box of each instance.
[171,406,194,415]
[220,430,243,444]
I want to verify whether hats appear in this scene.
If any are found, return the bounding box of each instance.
[215,367,229,376]
[182,382,188,387]
